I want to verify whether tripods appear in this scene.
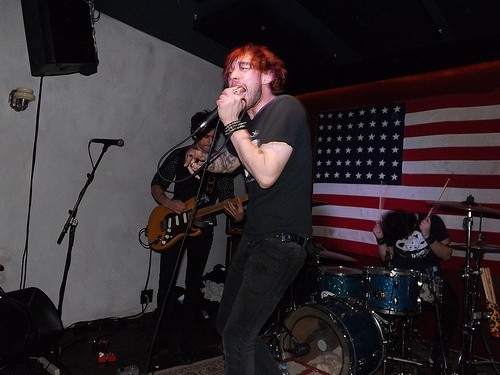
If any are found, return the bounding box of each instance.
[431,194,500,375]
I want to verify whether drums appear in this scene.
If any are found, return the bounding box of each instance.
[411,273,441,312]
[278,295,385,375]
[364,267,409,315]
[319,265,362,298]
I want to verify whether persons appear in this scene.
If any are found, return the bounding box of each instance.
[150,110,244,310]
[373,209,453,336]
[184,44,311,375]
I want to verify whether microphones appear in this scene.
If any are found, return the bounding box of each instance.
[200,86,246,127]
[92,138,124,147]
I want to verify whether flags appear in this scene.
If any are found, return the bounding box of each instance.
[311,91,500,293]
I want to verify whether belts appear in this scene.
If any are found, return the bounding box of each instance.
[275,232,309,248]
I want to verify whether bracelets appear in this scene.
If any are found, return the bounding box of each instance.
[425,234,435,245]
[224,120,247,136]
[377,236,385,246]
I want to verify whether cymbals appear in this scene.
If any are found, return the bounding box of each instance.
[425,201,500,214]
[445,242,500,250]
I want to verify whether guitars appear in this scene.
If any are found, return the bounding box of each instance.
[147,192,248,253]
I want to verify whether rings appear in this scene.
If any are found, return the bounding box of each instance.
[233,89,240,95]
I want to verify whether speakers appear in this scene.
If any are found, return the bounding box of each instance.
[225,229,294,334]
[225,212,246,235]
[21,0,100,77]
[0,286,64,368]
